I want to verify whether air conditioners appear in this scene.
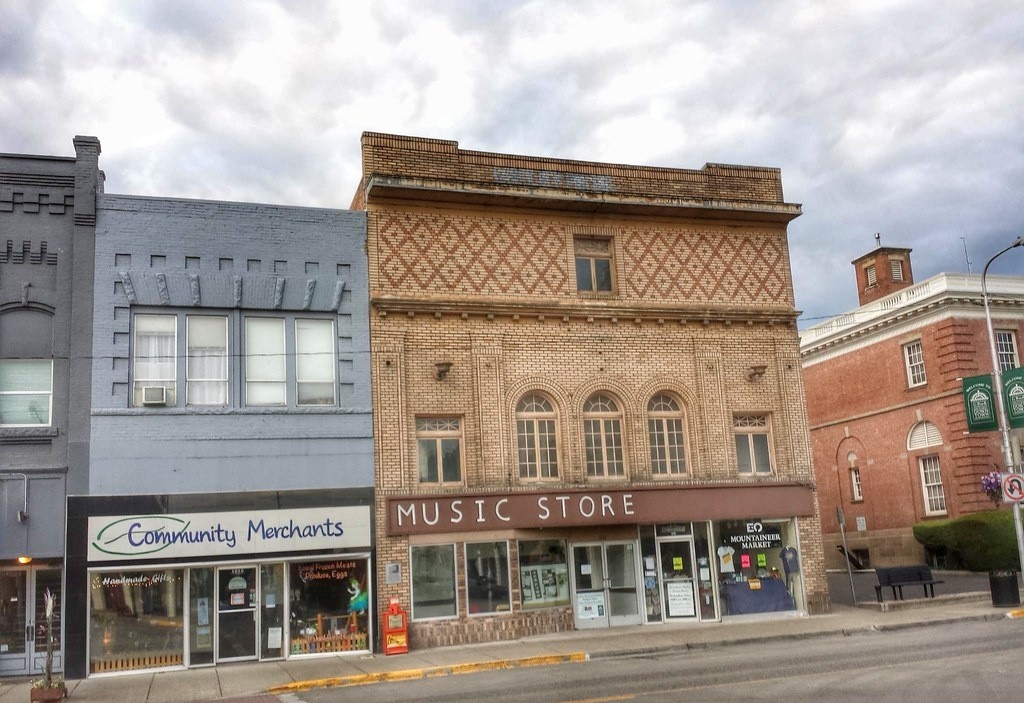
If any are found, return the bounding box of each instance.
[142,386,167,405]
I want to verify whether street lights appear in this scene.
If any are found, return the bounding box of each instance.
[980,235,1024,589]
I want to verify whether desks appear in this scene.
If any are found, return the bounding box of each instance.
[721,577,793,617]
[307,614,367,636]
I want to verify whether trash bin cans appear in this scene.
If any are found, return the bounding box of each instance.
[988,568,1021,608]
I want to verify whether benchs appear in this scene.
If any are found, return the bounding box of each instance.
[874,564,945,603]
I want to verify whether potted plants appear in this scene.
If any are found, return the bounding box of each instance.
[29,587,68,703]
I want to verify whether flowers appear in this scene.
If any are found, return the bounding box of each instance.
[980,470,1003,508]
[30,677,65,689]
